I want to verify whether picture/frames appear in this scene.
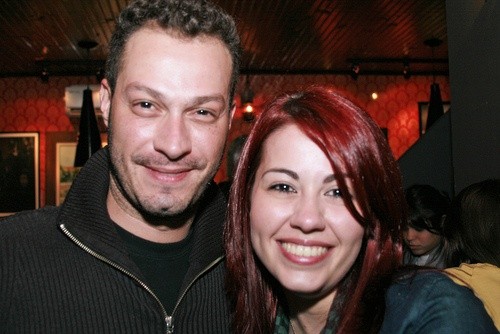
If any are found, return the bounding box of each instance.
[45,131,107,207]
[418,102,453,137]
[0,132,40,217]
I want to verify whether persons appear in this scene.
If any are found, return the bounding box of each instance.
[224,87,498,334]
[442,184,500,331]
[0,0,241,334]
[404,184,444,268]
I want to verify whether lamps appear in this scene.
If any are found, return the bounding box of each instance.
[240,60,257,126]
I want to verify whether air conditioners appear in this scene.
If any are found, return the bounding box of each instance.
[65,86,104,116]
